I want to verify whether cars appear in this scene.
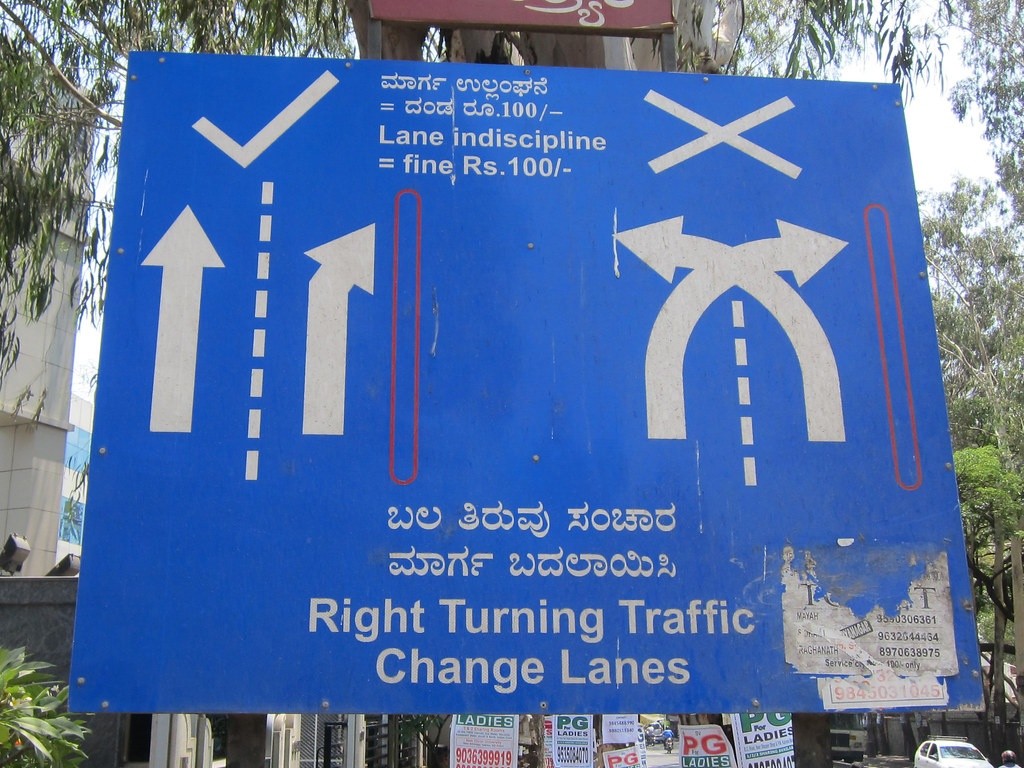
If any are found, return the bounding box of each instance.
[915,739,995,768]
[649,718,666,743]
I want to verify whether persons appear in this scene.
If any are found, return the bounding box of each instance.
[646,724,655,736]
[662,726,674,748]
[998,750,1022,768]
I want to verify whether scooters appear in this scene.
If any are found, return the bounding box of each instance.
[664,736,674,754]
[645,733,656,746]
